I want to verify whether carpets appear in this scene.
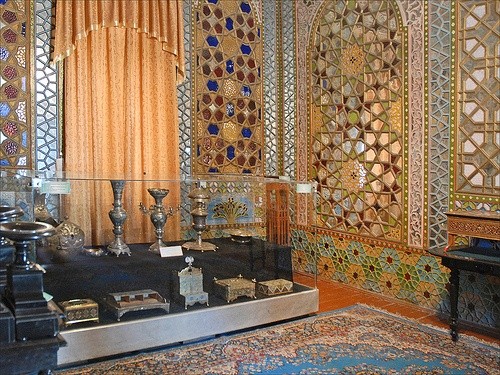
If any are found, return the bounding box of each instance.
[55,301,500,375]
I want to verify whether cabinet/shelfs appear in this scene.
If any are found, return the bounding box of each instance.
[5,173,322,369]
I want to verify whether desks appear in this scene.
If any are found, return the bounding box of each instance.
[423,245,500,344]
